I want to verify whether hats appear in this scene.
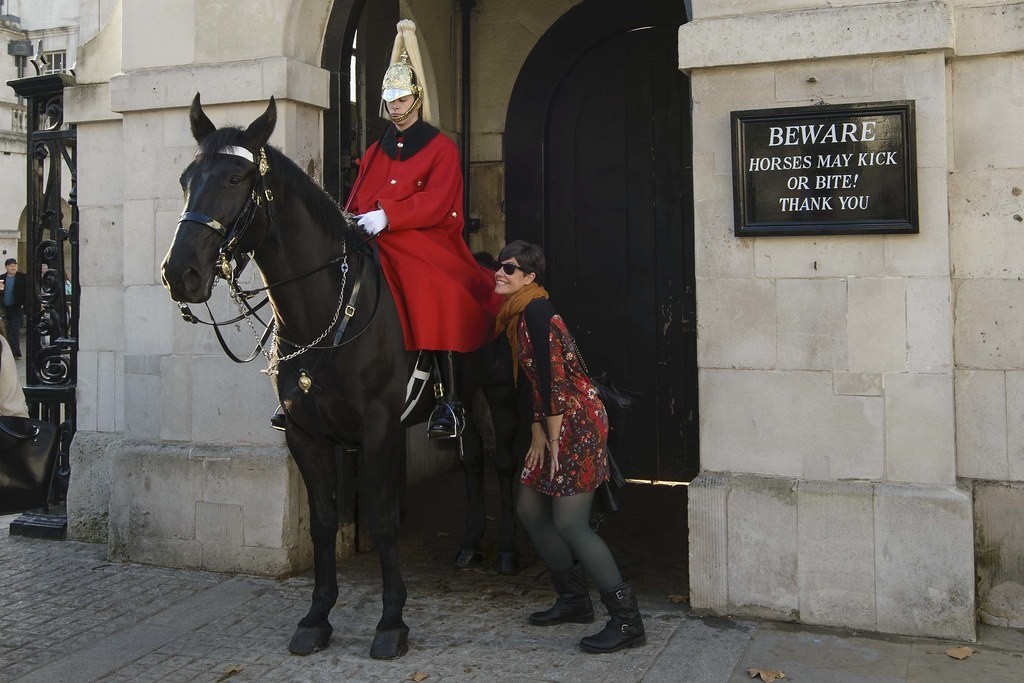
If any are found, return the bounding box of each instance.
[379,19,423,121]
[5,259,17,265]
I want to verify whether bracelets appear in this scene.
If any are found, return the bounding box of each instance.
[549,438,559,442]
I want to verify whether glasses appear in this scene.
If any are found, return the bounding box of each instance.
[489,259,530,276]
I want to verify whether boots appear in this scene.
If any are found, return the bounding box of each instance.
[529,560,595,627]
[579,581,647,655]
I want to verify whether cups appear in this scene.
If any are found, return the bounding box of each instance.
[0,280,4,290]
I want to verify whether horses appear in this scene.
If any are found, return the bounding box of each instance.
[159,89,525,662]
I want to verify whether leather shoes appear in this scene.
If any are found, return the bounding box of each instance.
[272,413,287,431]
[428,405,468,437]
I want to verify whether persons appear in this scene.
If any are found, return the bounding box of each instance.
[494,239,645,653]
[65,272,72,320]
[271,63,511,437]
[0,258,27,360]
[0,335,29,417]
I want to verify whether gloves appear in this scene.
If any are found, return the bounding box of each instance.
[353,207,389,235]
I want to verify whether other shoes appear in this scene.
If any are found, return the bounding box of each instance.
[14,355,23,361]
